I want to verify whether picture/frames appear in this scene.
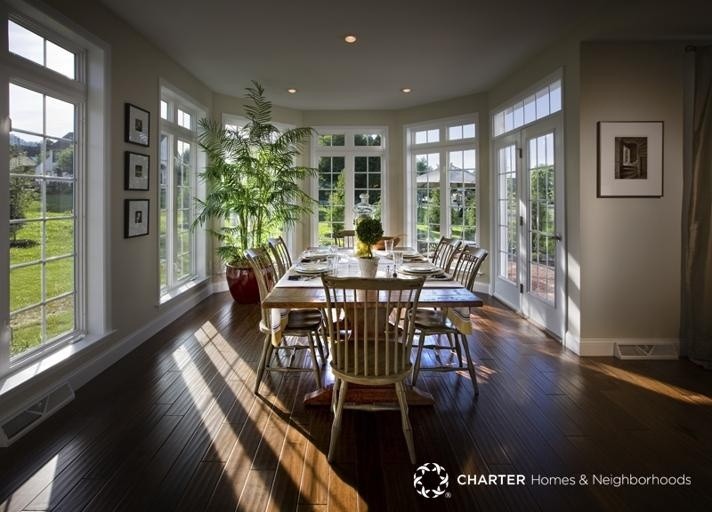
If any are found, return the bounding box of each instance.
[124,198,149,238]
[125,102,150,147]
[596,120,664,198]
[124,151,150,191]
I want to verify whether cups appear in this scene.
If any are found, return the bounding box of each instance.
[326,239,403,277]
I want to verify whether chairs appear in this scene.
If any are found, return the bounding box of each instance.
[267,237,329,356]
[334,229,356,247]
[243,245,326,394]
[409,245,488,395]
[410,236,462,352]
[321,272,426,464]
[383,237,400,248]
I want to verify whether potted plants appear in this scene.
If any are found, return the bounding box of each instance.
[357,218,384,278]
[190,80,322,305]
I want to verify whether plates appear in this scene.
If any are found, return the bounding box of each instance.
[295,246,441,276]
